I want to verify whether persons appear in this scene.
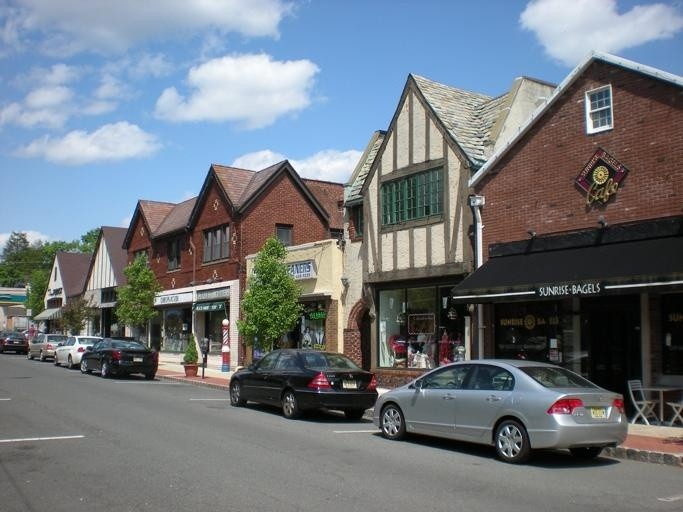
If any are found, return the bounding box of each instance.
[28,324,35,338]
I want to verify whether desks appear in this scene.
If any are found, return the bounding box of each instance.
[632,385,682,425]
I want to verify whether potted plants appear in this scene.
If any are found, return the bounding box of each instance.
[180,333,200,376]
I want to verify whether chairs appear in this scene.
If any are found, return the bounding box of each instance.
[626,378,660,426]
[473,368,493,389]
[665,399,682,427]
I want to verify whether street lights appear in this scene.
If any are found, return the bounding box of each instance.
[24,283,31,340]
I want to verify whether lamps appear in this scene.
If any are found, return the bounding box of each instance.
[340,277,349,289]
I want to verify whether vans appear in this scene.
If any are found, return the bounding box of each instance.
[26,333,68,362]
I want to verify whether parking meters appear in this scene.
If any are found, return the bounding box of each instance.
[199,337,208,379]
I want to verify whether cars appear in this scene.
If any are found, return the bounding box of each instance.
[229,348,378,419]
[161,328,221,352]
[0,330,28,355]
[14,328,44,340]
[52,335,103,369]
[78,336,158,380]
[372,359,628,462]
